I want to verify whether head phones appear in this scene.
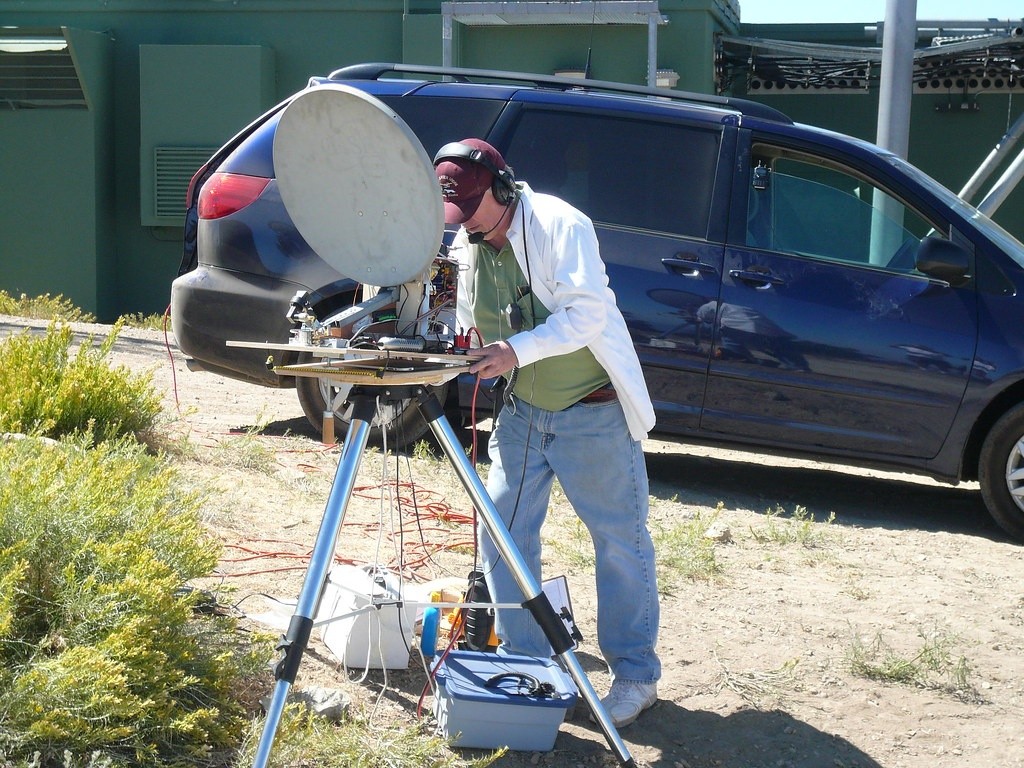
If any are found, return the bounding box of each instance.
[429,142,516,207]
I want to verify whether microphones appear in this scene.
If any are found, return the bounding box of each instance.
[468,196,513,244]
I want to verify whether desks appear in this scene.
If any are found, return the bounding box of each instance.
[254,357,634,768]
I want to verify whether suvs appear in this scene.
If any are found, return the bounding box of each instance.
[169,64,1024,542]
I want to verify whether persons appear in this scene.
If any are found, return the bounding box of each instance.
[434,139,659,727]
[697,298,812,373]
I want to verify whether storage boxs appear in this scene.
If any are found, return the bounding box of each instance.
[429,650,578,751]
[317,565,417,670]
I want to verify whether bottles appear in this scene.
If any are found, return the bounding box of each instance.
[322,411,334,444]
[421,592,441,657]
